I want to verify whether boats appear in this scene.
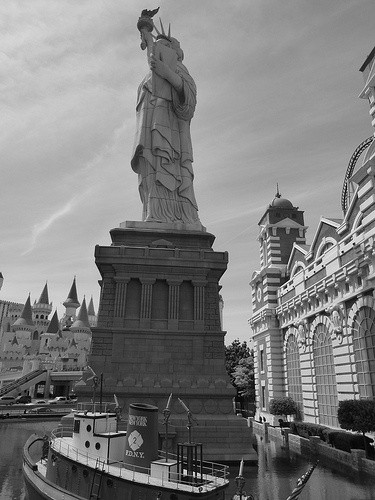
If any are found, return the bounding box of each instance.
[20,372,231,500]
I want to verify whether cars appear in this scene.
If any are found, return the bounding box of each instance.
[29,400,51,405]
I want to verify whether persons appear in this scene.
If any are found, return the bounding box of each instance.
[127,5,204,228]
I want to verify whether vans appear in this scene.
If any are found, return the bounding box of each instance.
[16,395,32,404]
[0,396,16,405]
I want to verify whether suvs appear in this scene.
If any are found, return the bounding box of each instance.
[49,396,69,404]
